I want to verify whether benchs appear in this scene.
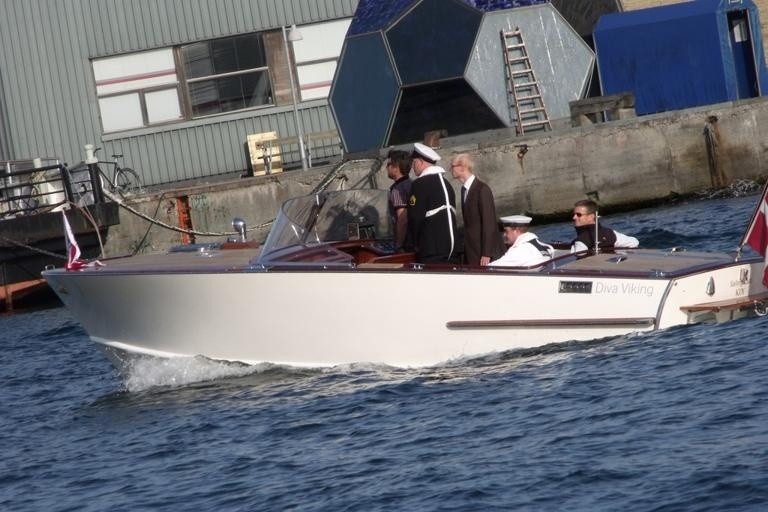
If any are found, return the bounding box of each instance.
[255,128,344,175]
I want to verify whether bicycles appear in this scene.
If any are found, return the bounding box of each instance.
[94,148,141,197]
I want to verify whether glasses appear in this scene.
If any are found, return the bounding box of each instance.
[450,164,462,167]
[572,212,591,217]
[387,162,392,166]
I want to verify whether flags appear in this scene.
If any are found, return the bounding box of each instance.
[59,212,106,271]
[750,190,768,290]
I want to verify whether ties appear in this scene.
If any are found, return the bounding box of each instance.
[461,186,466,207]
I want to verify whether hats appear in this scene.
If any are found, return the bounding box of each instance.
[408,142,442,164]
[500,214,532,227]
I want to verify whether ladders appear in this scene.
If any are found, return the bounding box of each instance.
[500,25,553,137]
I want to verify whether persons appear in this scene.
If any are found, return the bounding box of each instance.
[570,199,639,257]
[488,215,556,271]
[452,153,507,269]
[407,142,459,263]
[386,149,411,252]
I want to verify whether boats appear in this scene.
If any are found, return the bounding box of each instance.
[40,154,767,392]
[1,145,120,311]
